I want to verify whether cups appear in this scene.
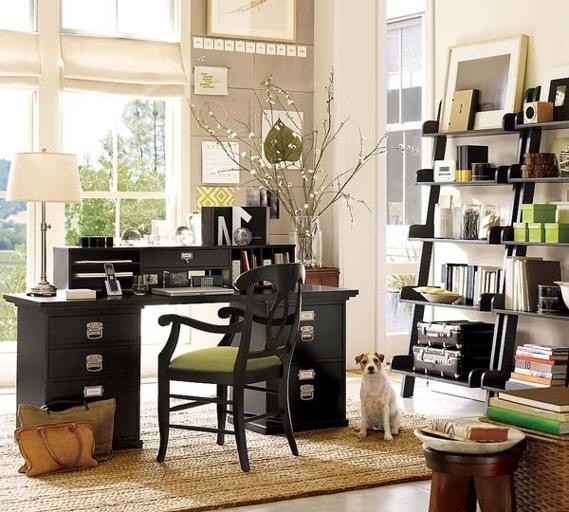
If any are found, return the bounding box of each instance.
[200,277,215,291]
[133,274,150,297]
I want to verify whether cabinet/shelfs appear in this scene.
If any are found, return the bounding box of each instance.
[386,34,568,397]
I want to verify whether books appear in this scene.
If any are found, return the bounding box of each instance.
[489,397,569,422]
[497,385,569,413]
[486,407,569,435]
[432,417,509,442]
[505,343,569,389]
[504,257,561,311]
[441,263,504,306]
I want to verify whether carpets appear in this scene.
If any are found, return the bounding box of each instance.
[0,393,440,512]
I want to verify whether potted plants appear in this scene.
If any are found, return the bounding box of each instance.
[185,70,418,269]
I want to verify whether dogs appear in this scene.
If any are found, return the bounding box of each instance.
[354,353,402,443]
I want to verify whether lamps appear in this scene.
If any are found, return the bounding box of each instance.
[5,152,78,297]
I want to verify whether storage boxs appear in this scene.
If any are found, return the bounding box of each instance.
[478,416,569,510]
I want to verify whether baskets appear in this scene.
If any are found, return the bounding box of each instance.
[513,430,569,512]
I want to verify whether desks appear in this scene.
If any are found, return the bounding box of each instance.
[2,283,359,452]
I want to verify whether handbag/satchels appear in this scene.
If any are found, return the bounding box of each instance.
[14,396,117,473]
[13,421,98,478]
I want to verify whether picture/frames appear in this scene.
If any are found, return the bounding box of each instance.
[206,0,299,42]
[193,66,226,96]
[437,35,526,131]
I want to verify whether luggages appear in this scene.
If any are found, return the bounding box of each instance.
[413,319,495,351]
[409,344,492,380]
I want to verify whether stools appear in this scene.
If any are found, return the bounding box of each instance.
[421,440,524,511]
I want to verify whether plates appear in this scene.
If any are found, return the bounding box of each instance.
[412,421,527,457]
[421,291,461,306]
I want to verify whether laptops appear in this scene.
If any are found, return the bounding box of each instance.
[151,287,235,297]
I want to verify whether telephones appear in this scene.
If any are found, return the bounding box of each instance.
[102,264,121,295]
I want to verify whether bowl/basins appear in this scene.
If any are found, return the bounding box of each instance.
[553,281,569,311]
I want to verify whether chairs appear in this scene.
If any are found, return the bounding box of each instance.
[153,261,307,474]
